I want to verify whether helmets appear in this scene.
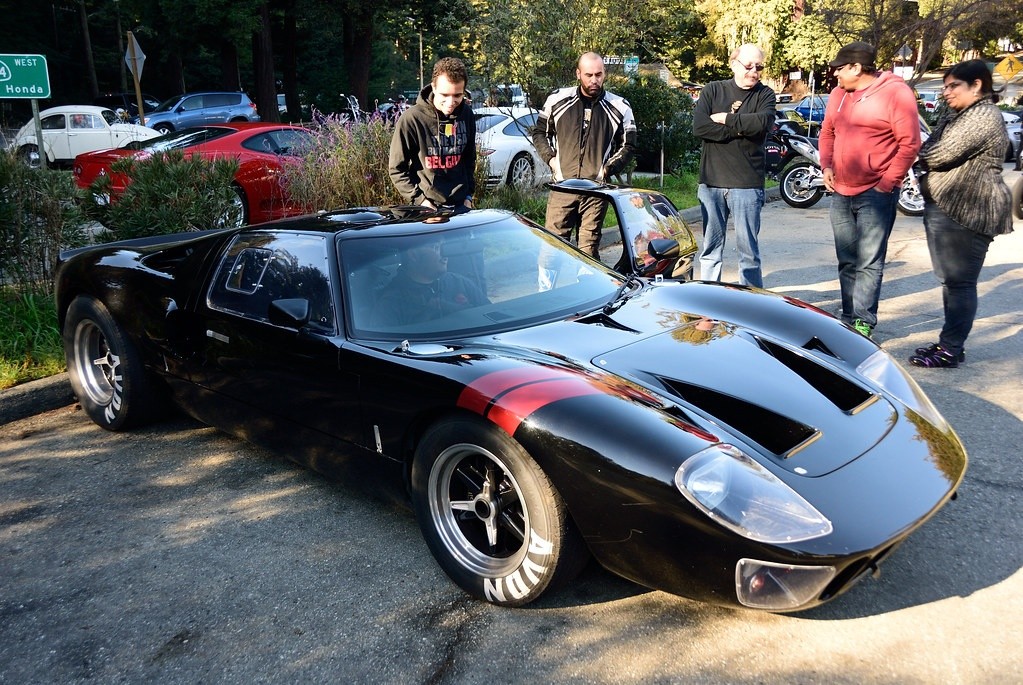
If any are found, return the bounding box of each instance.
[116,108,125,117]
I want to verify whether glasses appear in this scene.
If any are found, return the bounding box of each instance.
[940,80,965,92]
[736,58,764,71]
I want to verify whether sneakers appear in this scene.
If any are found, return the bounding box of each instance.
[915,342,965,362]
[908,345,958,368]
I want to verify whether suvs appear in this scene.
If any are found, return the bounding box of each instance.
[131,90,262,135]
[91,92,164,117]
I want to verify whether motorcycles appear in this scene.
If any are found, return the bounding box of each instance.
[762,116,925,217]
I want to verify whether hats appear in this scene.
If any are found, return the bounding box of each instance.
[829,42,877,67]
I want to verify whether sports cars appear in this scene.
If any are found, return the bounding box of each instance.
[52,178,969,609]
[71,121,374,233]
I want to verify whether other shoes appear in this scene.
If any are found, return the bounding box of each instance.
[853,319,871,338]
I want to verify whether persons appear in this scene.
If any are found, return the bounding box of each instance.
[818,42,922,338]
[369,237,493,328]
[693,44,776,288]
[630,194,676,216]
[117,108,135,124]
[532,52,637,293]
[635,231,668,266]
[388,57,477,212]
[909,60,1014,368]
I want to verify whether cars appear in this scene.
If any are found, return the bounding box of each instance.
[8,105,165,168]
[277,93,309,115]
[376,83,533,128]
[472,106,557,193]
[764,89,1023,162]
